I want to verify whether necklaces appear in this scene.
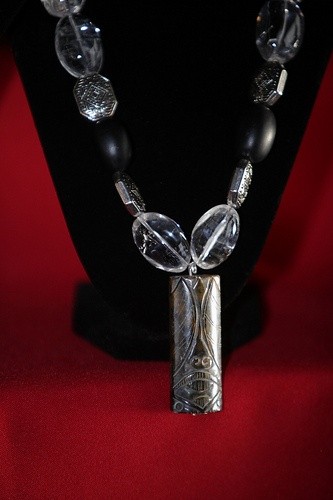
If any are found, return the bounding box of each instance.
[38,0,303,414]
